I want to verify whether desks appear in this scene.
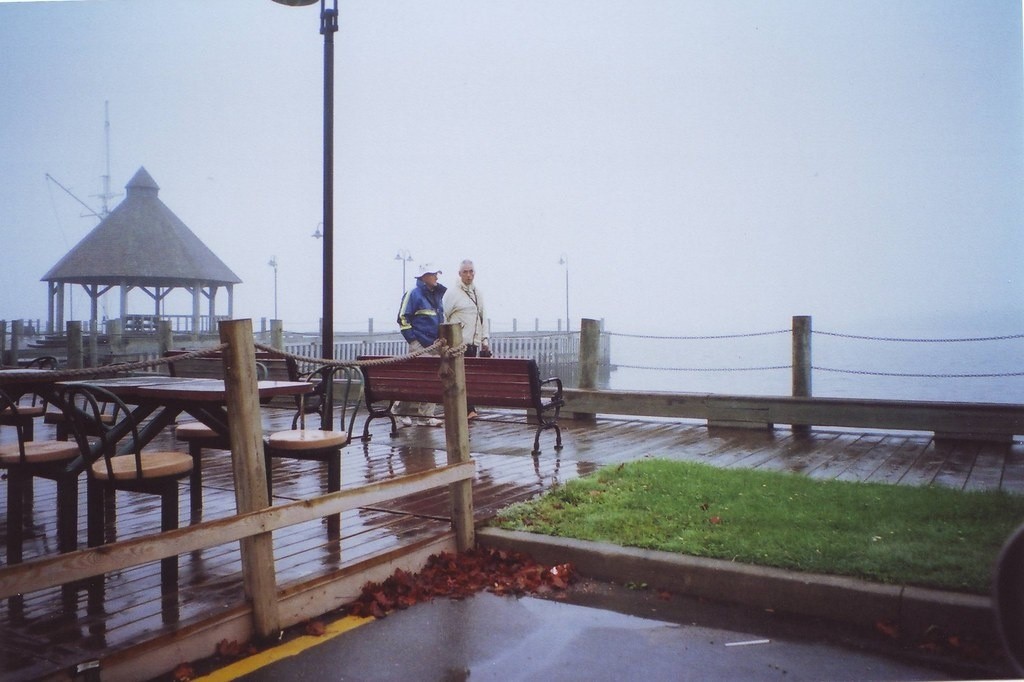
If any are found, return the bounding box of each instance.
[54,376,314,477]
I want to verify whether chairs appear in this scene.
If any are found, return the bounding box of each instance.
[0,357,193,583]
[176,421,270,509]
[268,363,364,540]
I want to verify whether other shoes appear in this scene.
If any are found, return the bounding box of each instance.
[468,410,479,421]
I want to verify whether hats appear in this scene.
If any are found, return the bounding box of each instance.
[414,262,442,278]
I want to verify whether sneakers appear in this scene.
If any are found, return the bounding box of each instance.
[417,417,444,427]
[390,409,412,426]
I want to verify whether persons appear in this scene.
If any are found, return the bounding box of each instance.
[391,262,447,426]
[442,261,489,419]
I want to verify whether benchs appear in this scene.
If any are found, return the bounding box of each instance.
[164,350,333,430]
[356,355,565,456]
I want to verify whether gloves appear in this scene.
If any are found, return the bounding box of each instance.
[410,341,422,352]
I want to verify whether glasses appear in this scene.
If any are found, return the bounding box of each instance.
[424,273,437,277]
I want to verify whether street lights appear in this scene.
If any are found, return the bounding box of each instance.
[394,247,415,296]
[557,251,570,336]
[269,254,280,319]
